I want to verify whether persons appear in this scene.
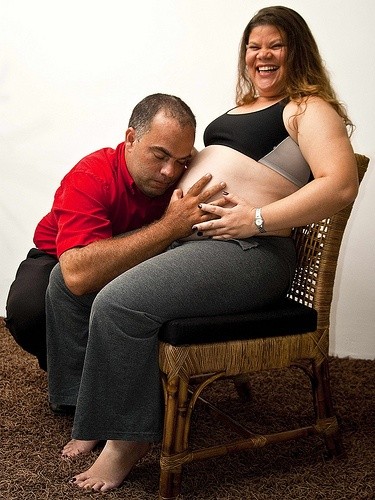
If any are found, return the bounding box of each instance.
[5,93,231,415]
[46,6,359,493]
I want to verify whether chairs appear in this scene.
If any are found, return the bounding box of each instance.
[154,152,372,498]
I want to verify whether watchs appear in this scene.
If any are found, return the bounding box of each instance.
[254,208,267,233]
